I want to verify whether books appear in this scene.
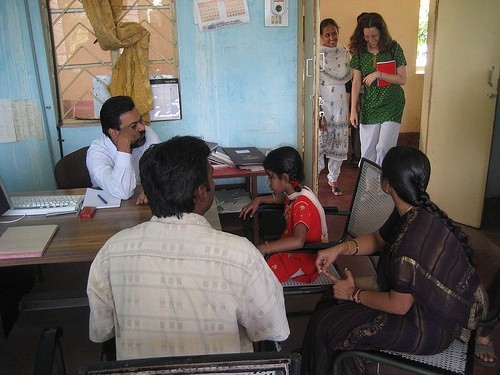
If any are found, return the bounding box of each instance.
[0,224,59,259]
[376,60,396,88]
[207,141,271,172]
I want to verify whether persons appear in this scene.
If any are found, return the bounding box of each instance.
[87,96,162,204]
[86,135,290,362]
[302,145,480,375]
[318,18,353,196]
[473,267,500,367]
[239,146,329,284]
[350,12,406,169]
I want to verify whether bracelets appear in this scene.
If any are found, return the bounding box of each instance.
[272,193,276,200]
[352,287,364,304]
[346,238,359,255]
[265,241,270,255]
[380,71,382,78]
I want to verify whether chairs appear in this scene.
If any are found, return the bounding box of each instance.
[53,145,94,190]
[86,336,303,375]
[331,268,500,375]
[32,326,67,375]
[243,157,397,353]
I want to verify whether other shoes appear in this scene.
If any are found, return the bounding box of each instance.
[350,154,359,166]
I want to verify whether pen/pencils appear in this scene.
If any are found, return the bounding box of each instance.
[97,193,107,204]
[75,204,82,218]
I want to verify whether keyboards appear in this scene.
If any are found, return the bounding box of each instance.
[3,195,85,216]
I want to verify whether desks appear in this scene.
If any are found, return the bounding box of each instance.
[0,184,223,341]
[208,161,269,247]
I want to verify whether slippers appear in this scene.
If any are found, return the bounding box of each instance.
[327,181,343,196]
[474,334,497,367]
